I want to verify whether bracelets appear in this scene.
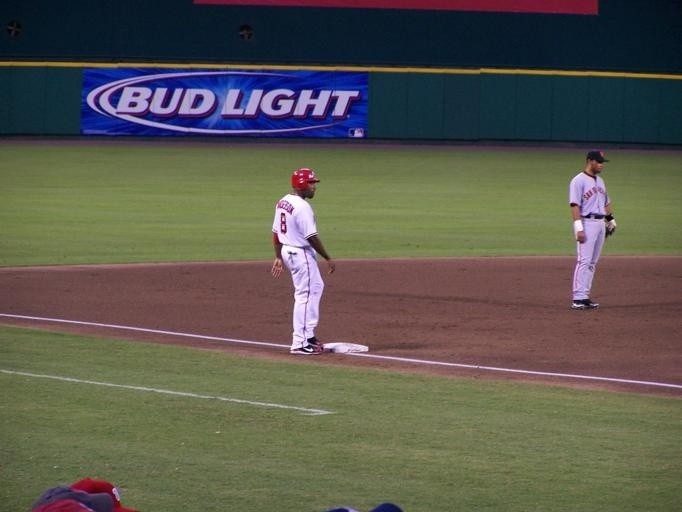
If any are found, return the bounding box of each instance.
[573,219,583,232]
[605,213,614,222]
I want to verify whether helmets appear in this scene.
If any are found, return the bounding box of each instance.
[290,166,321,190]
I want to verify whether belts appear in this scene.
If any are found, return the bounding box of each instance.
[585,214,605,220]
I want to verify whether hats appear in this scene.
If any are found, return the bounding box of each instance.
[32,486,116,511]
[587,150,610,163]
[70,477,139,512]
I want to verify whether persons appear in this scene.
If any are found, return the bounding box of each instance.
[270,168,338,355]
[568,150,617,311]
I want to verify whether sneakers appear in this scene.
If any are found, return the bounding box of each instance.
[307,336,325,351]
[288,345,322,356]
[571,299,600,309]
